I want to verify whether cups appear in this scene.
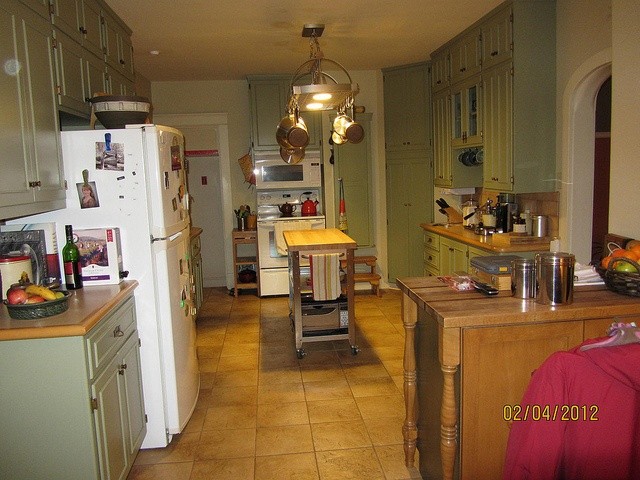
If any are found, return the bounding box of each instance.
[247,215,256,229]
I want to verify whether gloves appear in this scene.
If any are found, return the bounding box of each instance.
[238,154,253,182]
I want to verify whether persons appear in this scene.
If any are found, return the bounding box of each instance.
[80,184,97,208]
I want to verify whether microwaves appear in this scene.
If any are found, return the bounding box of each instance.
[255,151,322,190]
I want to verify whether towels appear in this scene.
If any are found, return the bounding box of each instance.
[309,252,342,301]
[273,220,312,256]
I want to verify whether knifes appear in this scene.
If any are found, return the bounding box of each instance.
[436,198,450,216]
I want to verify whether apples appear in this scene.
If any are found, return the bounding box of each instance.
[625,239,640,257]
[612,261,626,271]
[601,255,615,269]
[26,295,45,305]
[623,262,638,273]
[7,289,27,305]
[608,249,626,257]
[618,250,637,261]
[55,292,64,299]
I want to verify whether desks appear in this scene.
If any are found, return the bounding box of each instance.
[283,228,359,358]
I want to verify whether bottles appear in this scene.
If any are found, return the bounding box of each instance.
[62,225,83,290]
[525,210,532,235]
[550,236,559,252]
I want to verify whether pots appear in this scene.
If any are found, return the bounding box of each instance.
[462,149,476,166]
[84,95,151,129]
[239,268,256,282]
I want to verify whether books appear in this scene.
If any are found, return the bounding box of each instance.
[574,262,605,286]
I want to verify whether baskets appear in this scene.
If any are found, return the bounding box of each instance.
[596,255,640,297]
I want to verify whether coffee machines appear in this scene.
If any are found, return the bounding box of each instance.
[497,193,519,233]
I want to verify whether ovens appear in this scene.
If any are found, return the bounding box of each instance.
[256,221,325,297]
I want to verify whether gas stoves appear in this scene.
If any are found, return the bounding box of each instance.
[258,210,325,220]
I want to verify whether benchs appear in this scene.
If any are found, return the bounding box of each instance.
[341,255,377,273]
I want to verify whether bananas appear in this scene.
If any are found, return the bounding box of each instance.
[24,284,57,301]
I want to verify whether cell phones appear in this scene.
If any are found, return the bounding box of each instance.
[472,280,499,295]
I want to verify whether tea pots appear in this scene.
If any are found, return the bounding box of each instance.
[300,191,319,216]
[277,202,296,215]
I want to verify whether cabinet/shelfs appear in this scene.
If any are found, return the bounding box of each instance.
[52,0,104,58]
[1,280,148,480]
[480,5,513,73]
[102,0,133,74]
[431,48,450,94]
[105,66,134,97]
[54,39,105,116]
[380,60,431,152]
[393,272,640,480]
[231,228,260,298]
[436,235,468,281]
[16,0,51,23]
[245,70,321,151]
[189,226,205,311]
[481,62,516,193]
[420,223,440,279]
[466,244,500,278]
[450,72,484,150]
[429,87,455,188]
[384,156,434,283]
[0,6,66,222]
[449,19,481,85]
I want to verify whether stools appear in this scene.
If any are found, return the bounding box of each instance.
[353,272,381,297]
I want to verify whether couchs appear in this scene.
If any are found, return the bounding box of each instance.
[500,320,640,480]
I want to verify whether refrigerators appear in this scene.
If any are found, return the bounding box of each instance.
[6,122,202,449]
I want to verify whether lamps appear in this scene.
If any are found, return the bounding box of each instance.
[275,22,365,165]
